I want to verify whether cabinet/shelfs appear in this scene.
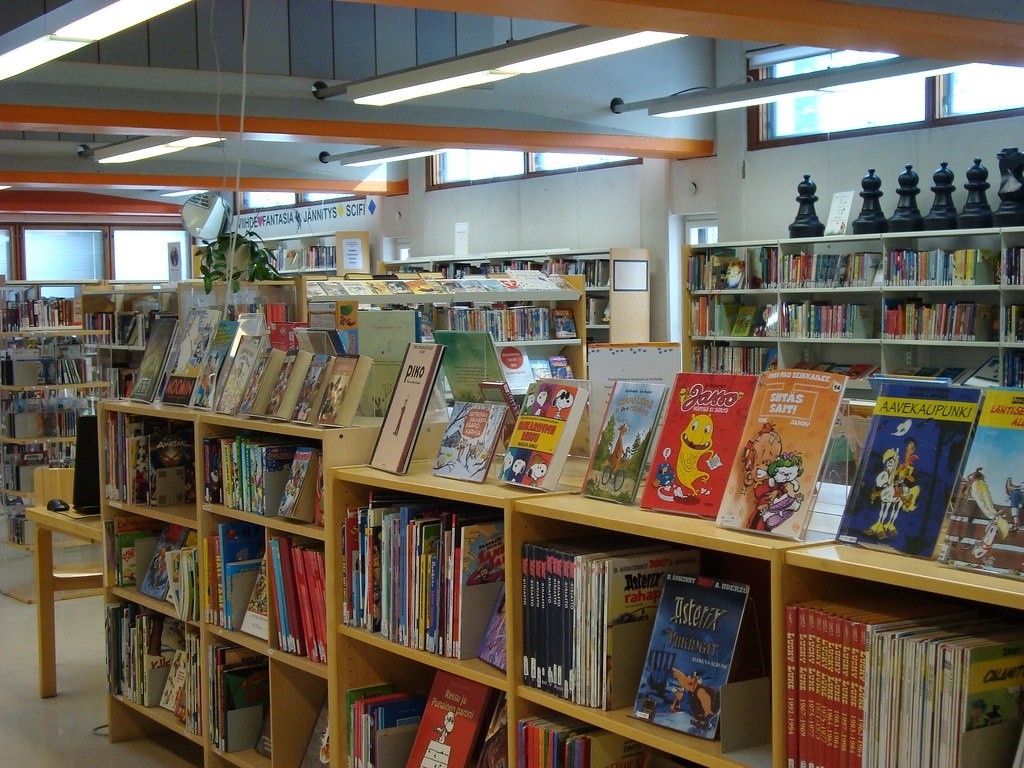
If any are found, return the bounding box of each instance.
[1,225,1024,768]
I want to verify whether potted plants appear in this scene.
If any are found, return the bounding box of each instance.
[194,211,285,293]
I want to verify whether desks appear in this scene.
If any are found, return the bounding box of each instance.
[24,505,103,699]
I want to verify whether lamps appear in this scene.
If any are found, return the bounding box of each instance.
[646,56,978,118]
[0,0,193,80]
[93,136,228,164]
[340,146,449,166]
[347,22,689,106]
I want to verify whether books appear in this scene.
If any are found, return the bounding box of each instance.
[0,244,1024,768]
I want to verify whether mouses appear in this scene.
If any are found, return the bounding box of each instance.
[47,499,71,511]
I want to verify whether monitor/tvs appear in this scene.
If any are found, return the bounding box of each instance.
[72,414,99,516]
